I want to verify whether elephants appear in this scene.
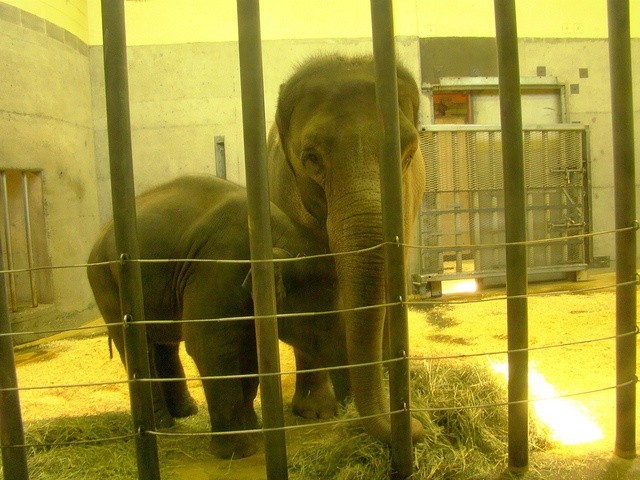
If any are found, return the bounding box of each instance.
[265,54,425,445]
[87,173,341,461]
